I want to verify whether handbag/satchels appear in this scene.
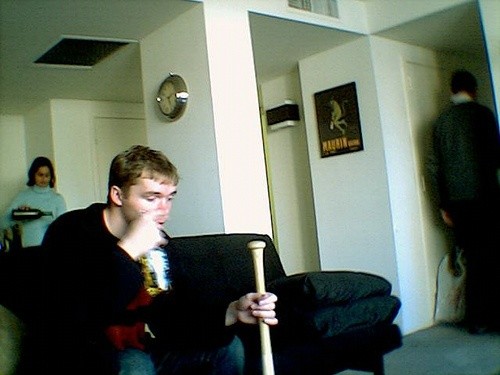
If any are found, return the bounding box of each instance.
[434,225,467,324]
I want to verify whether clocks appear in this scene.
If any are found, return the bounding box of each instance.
[155,72,189,122]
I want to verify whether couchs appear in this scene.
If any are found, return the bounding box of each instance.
[1,234,402,375]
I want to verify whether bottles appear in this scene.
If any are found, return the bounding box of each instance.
[11,208,54,221]
[126,220,180,297]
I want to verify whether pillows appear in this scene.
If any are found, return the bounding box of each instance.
[266,270,393,305]
[274,296,401,341]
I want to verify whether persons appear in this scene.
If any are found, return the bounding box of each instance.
[14,144,279,375]
[423,69,500,337]
[327,99,347,136]
[0,157,68,251]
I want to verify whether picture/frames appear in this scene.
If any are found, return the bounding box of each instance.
[313,82,364,158]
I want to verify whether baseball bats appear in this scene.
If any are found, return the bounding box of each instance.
[247,240,275,375]
